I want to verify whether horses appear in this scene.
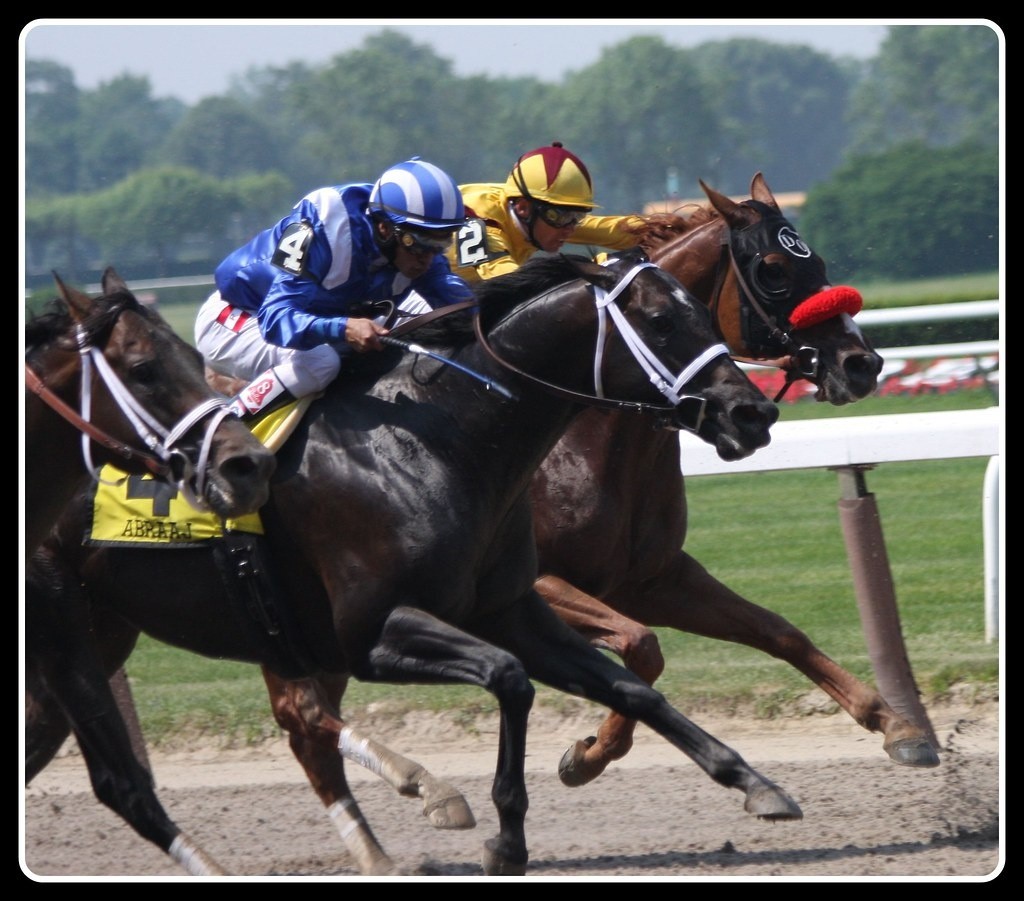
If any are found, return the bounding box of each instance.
[16,170,942,875]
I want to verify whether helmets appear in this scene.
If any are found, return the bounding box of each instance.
[367,154,469,241]
[503,141,603,212]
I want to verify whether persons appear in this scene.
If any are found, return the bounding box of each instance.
[438,138,653,282]
[194,155,478,424]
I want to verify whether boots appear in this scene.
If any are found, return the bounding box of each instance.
[225,366,298,431]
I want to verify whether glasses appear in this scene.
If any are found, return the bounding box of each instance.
[405,228,449,257]
[532,200,589,228]
